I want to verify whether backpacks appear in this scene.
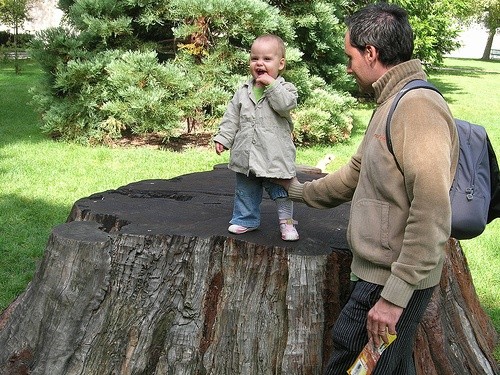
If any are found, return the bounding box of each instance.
[385,79,500,239]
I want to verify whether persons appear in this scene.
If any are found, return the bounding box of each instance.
[270,4,460,375]
[213,34,299,240]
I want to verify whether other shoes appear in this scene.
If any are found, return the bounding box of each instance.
[227,224,259,234]
[279,218,300,241]
